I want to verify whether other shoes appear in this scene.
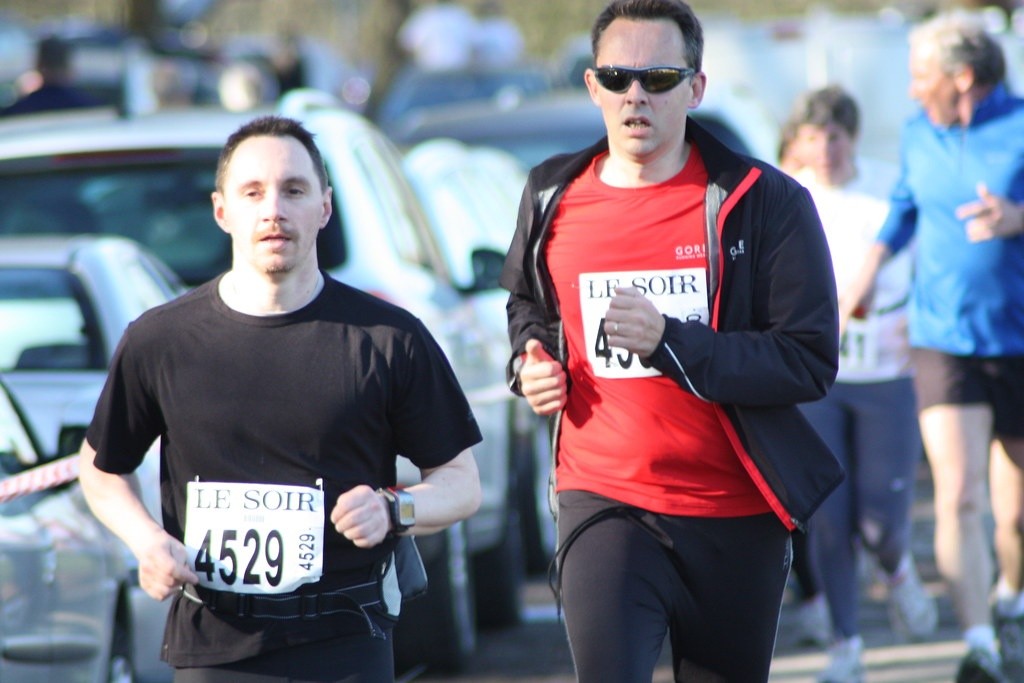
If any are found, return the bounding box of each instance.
[798,595,832,646]
[817,633,866,683]
[956,649,1001,683]
[875,551,940,636]
[990,585,1024,683]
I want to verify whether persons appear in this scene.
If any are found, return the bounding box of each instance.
[499,0,846,682]
[78,117,482,683]
[774,9,1024,683]
[0,39,305,116]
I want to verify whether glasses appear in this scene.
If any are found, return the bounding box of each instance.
[590,65,696,94]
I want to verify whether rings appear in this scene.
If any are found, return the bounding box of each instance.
[614,322,619,336]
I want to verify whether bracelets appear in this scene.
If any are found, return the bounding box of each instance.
[516,363,525,396]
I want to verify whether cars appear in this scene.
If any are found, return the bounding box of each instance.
[0,233,200,683]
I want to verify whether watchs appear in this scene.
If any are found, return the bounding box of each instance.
[375,487,416,534]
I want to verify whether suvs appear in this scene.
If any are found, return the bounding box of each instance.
[0,1,1023,671]
